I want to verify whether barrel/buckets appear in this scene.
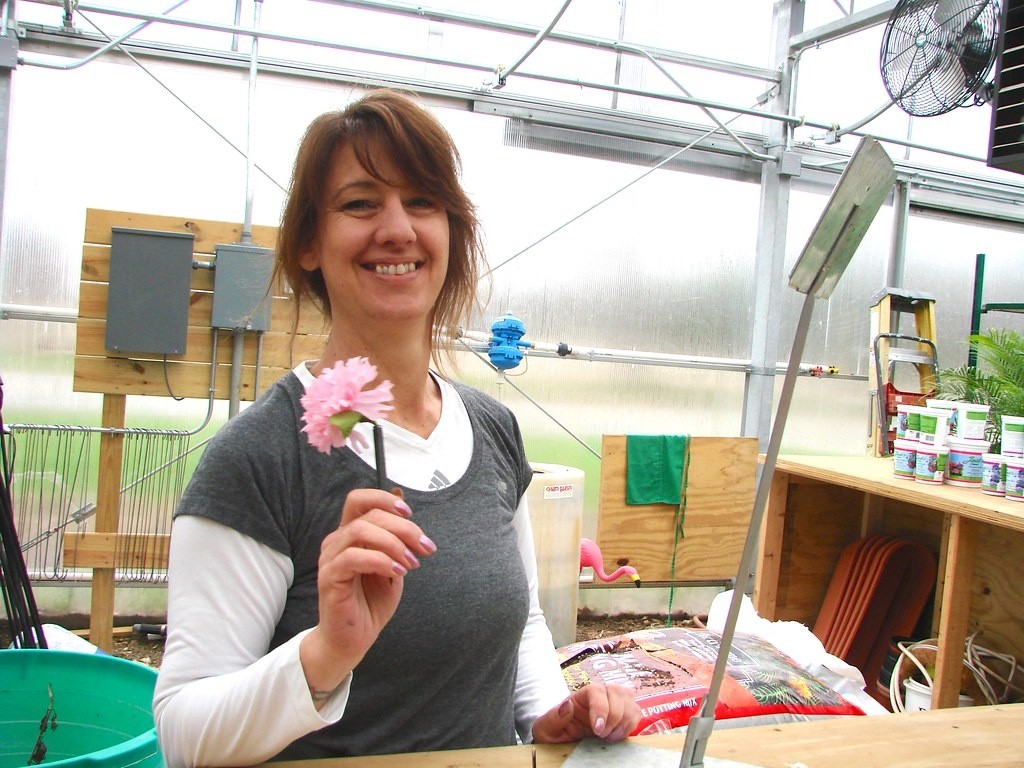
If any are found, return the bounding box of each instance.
[876,636,924,699]
[903,676,976,713]
[526,462,584,650]
[0,648,167,768]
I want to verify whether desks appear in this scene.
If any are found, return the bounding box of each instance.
[754,454,1024,710]
[253,705,1024,767]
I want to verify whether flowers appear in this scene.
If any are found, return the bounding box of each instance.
[300,356,395,587]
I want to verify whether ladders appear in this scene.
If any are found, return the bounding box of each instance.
[867,286,941,455]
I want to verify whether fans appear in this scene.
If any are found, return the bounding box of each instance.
[879,0,1002,117]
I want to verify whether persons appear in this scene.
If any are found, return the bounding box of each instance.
[151,90,644,767]
